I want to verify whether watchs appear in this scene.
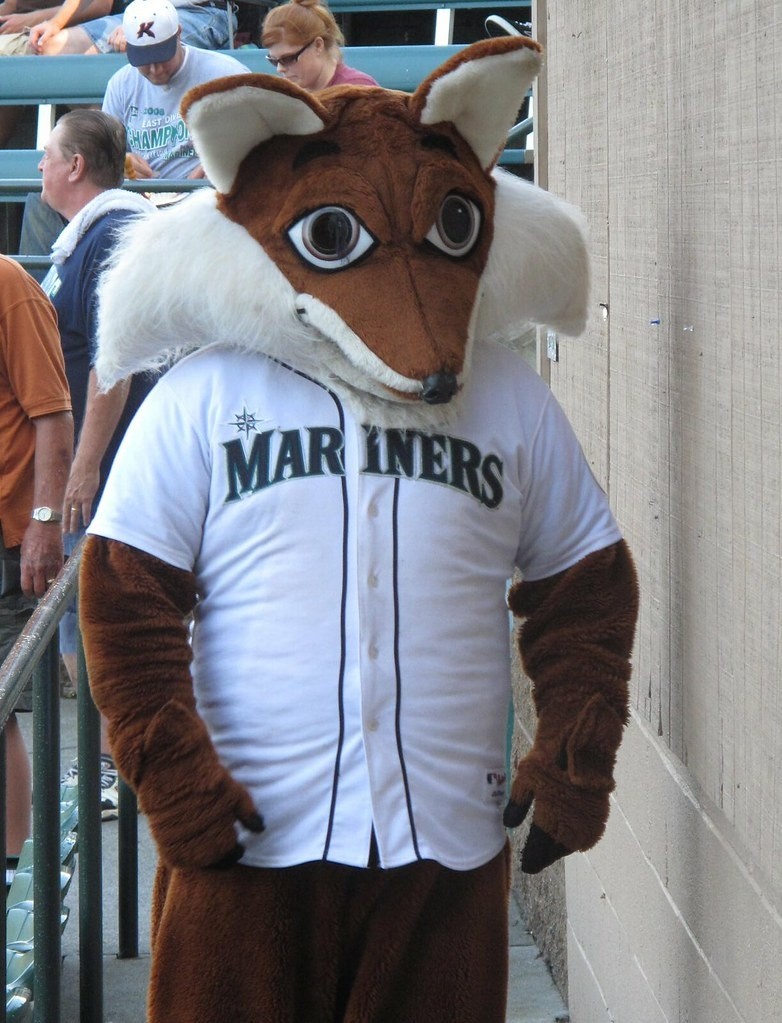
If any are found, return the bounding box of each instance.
[30,507,63,522]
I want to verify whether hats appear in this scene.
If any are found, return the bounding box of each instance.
[123,0,178,67]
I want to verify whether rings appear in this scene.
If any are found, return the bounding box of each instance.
[71,508,80,511]
[47,579,55,584]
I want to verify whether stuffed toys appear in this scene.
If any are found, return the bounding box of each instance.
[78,34,642,1023]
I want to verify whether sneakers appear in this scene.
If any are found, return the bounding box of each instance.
[99,766,142,823]
[61,753,114,786]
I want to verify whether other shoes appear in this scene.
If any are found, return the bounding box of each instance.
[59,677,76,700]
[5,855,21,888]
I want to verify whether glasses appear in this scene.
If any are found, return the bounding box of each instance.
[265,36,327,68]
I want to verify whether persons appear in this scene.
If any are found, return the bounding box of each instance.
[261,0,381,90]
[0,0,238,148]
[20,0,253,255]
[0,255,73,891]
[39,107,168,820]
